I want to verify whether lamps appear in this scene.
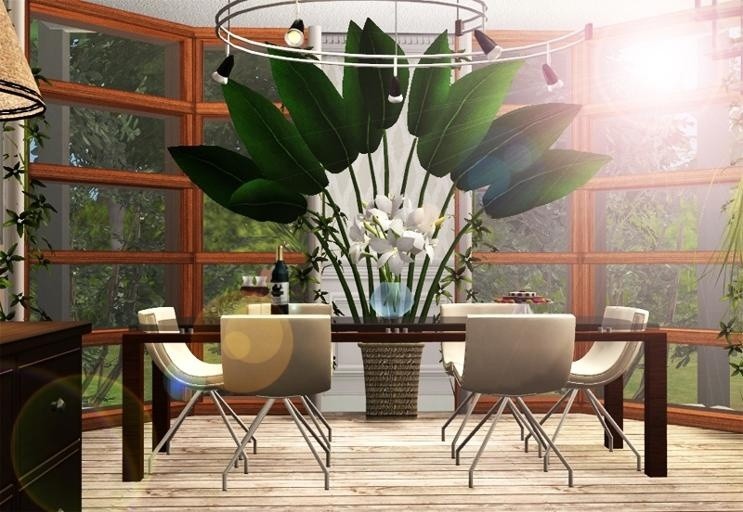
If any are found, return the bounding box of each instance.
[211,0,593,104]
[0,1,47,122]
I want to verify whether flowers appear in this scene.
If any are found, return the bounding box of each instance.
[169,17,614,371]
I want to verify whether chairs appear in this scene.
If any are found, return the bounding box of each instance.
[220,302,338,491]
[138,307,256,474]
[523,306,655,474]
[440,301,578,491]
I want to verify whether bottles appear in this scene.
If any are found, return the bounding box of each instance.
[271,246,290,315]
[240,276,268,297]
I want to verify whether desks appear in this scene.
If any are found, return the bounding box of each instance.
[123,317,666,481]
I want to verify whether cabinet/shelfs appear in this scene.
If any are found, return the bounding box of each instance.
[0,320,89,512]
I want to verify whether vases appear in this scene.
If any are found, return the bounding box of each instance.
[356,341,425,421]
